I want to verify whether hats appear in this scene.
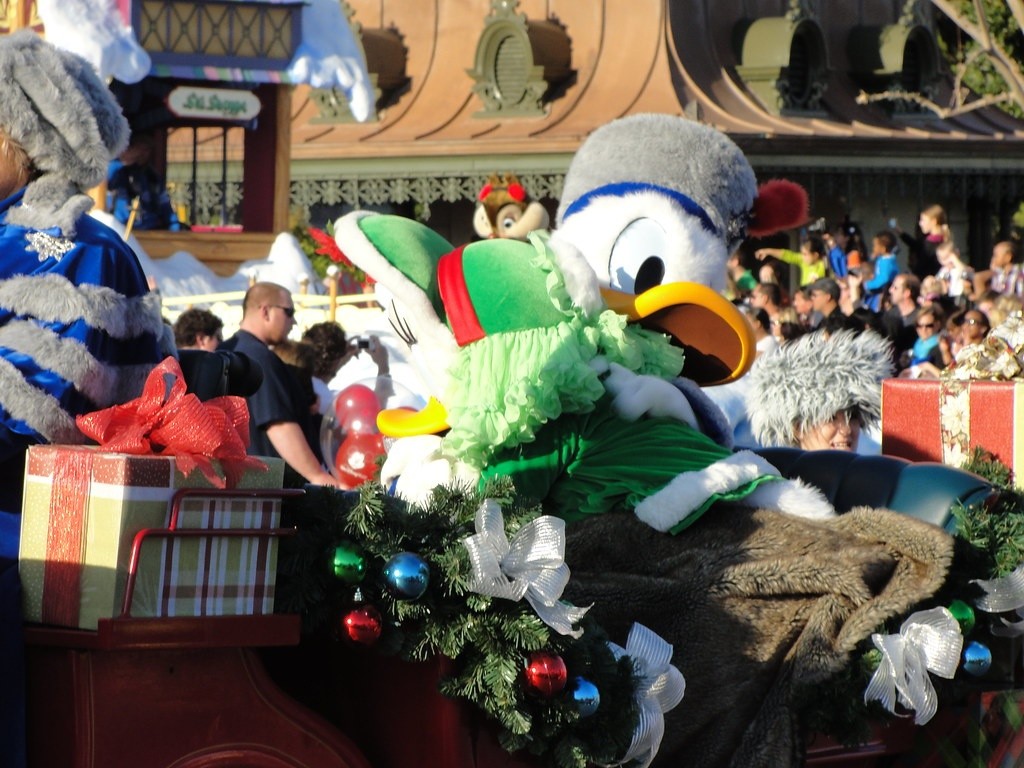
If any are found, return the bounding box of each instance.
[806,277,841,301]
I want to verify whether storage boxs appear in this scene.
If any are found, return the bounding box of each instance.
[879,376,1024,505]
[19,442,285,630]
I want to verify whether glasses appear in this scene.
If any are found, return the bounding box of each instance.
[257,304,295,317]
[914,320,938,330]
[963,318,984,327]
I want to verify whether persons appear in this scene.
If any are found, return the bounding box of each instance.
[220,283,344,490]
[0,31,175,584]
[719,194,1024,369]
[751,327,895,451]
[172,309,264,402]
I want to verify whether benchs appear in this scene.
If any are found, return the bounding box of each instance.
[727,445,1003,768]
[22,348,302,768]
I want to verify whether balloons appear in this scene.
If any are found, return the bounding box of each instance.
[318,380,429,488]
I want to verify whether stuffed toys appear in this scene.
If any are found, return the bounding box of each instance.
[335,105,833,523]
